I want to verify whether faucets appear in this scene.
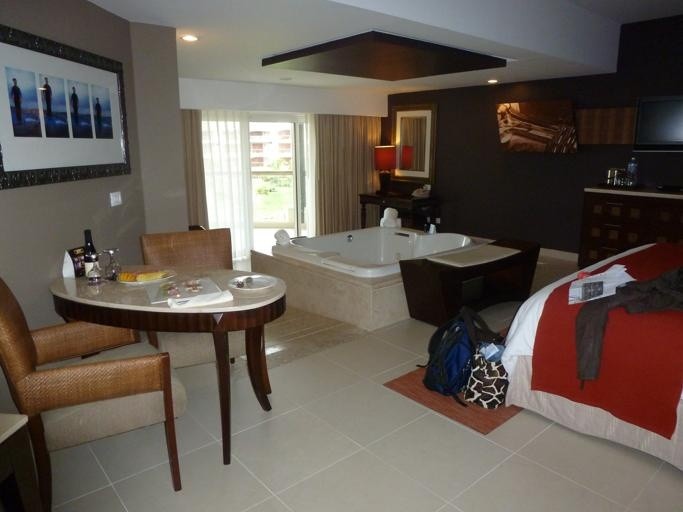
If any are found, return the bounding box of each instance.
[427,224,436,237]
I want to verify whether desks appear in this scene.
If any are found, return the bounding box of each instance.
[359,185,441,230]
[578,182,682,269]
[50,265,286,465]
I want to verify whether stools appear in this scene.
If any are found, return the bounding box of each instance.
[399,237,542,327]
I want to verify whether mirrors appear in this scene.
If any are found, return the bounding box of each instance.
[389,105,436,184]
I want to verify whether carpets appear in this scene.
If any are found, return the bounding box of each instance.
[381,364,526,435]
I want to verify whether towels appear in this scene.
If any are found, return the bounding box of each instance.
[168,289,234,309]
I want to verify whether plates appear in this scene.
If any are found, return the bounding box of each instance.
[228,275,276,290]
[117,270,176,284]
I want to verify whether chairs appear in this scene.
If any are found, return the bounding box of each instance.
[0,276,185,511]
[141,229,233,349]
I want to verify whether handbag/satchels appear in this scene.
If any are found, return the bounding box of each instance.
[416,310,477,406]
[464,307,510,409]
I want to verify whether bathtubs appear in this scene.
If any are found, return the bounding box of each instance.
[271,207,473,278]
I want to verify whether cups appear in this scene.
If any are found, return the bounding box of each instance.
[103,248,128,287]
[86,254,102,288]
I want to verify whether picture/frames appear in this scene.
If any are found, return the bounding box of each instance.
[0,23,130,191]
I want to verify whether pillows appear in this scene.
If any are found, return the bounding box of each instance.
[501,243,683,475]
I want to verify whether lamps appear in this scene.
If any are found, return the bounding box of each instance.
[375,146,395,195]
[402,144,414,170]
[181,34,198,42]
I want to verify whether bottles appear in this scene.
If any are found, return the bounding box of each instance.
[627,157,637,173]
[82,229,99,278]
